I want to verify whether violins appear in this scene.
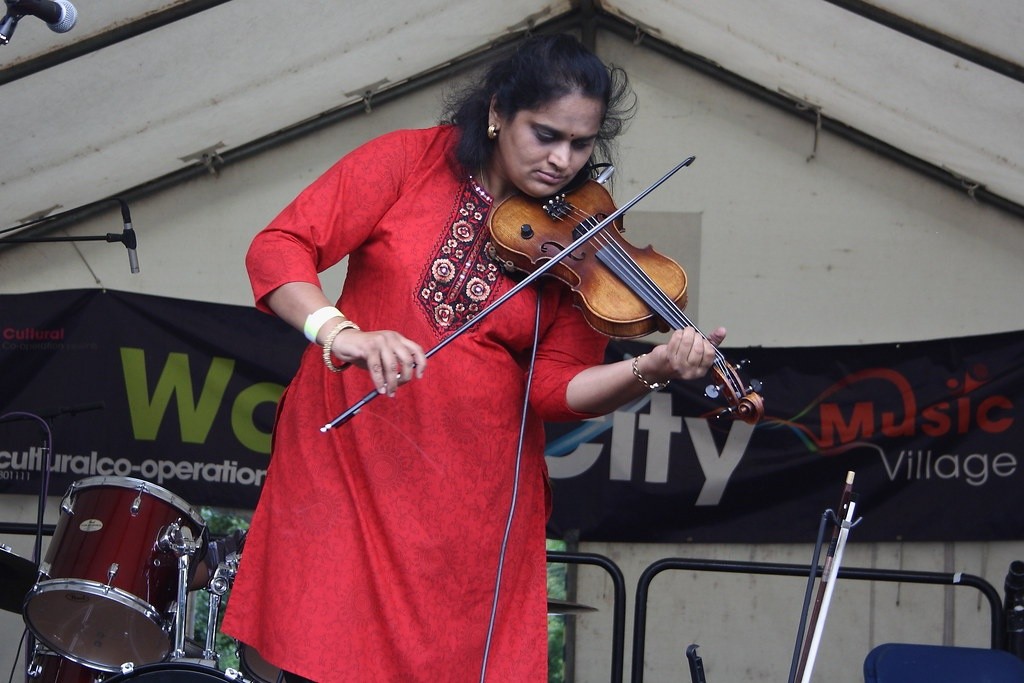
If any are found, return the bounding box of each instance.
[483,175,768,426]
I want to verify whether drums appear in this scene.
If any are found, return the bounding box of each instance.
[241,636,287,683]
[23,476,211,673]
[102,661,254,683]
[29,645,117,683]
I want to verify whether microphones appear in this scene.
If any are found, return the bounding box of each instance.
[119,202,140,273]
[4,0,77,33]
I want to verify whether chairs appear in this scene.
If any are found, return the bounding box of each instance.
[863,643,1024,683]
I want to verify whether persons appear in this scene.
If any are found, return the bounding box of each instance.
[220,30,726,683]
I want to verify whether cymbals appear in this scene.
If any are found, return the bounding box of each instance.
[0,548,38,615]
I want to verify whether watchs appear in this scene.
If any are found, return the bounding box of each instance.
[632,353,670,391]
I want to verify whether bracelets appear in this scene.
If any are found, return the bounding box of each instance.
[303,306,345,346]
[322,321,360,372]
